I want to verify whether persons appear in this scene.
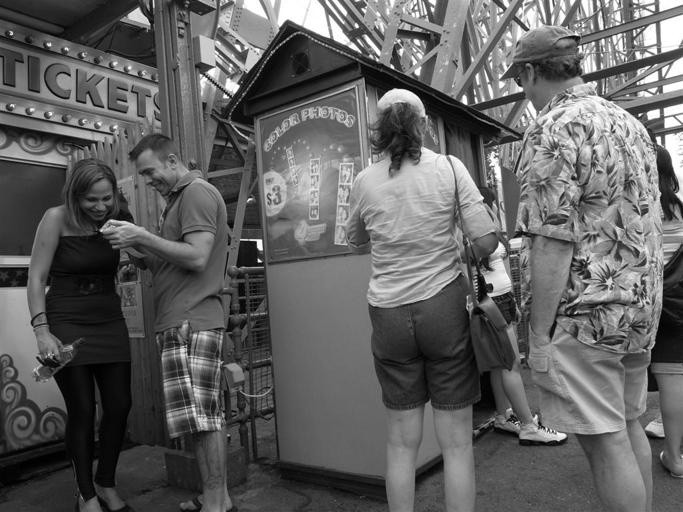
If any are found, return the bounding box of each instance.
[459,186,568,446]
[342,86,498,509]
[98,133,237,512]
[26,158,148,510]
[648,142,683,479]
[496,24,663,511]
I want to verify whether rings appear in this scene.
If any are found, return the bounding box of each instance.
[45,352,53,358]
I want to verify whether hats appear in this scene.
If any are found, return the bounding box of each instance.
[376,87,427,121]
[498,24,581,82]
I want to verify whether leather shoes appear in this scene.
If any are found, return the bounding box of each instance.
[92,479,136,512]
[75,492,104,512]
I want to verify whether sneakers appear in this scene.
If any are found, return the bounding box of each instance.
[492,407,523,438]
[519,413,569,446]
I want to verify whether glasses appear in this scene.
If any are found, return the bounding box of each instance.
[513,66,525,86]
[35,352,61,368]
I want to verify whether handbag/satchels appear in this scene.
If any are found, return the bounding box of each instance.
[468,294,517,377]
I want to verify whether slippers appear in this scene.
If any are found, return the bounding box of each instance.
[179,496,238,512]
[659,449,683,479]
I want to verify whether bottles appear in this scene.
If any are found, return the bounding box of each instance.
[30,337,86,385]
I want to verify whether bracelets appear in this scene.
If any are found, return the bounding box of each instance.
[33,322,50,331]
[30,311,48,327]
